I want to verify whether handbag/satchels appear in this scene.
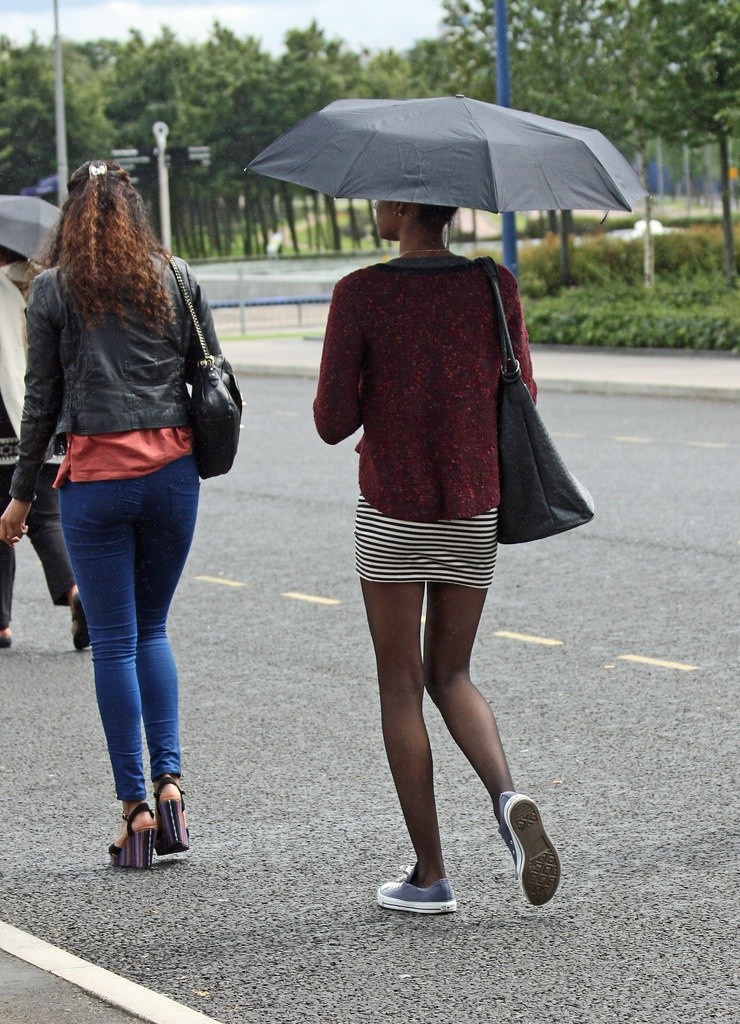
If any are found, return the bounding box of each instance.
[192,354,245,481]
[475,253,595,544]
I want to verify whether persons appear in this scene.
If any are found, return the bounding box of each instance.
[0,246,92,653]
[314,197,564,914]
[1,165,245,870]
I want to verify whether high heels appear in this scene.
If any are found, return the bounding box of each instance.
[106,776,188,866]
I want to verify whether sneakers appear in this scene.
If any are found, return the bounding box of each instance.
[376,791,563,914]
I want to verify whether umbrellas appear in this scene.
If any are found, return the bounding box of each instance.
[0,192,68,267]
[241,93,650,248]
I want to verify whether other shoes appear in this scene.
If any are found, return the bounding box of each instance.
[0,591,90,651]
